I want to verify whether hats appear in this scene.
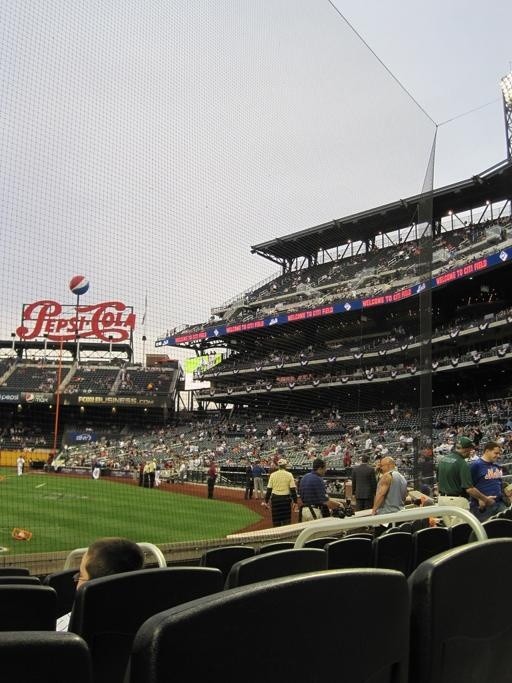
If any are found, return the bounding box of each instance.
[277,458,288,465]
[456,436,476,449]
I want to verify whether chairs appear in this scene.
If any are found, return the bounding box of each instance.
[1,506,512,681]
[1,355,170,471]
[171,221,511,475]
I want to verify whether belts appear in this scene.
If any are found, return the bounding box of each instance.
[439,494,465,497]
[305,504,324,508]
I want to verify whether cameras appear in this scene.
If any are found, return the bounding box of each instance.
[331,506,345,518]
[345,504,355,516]
